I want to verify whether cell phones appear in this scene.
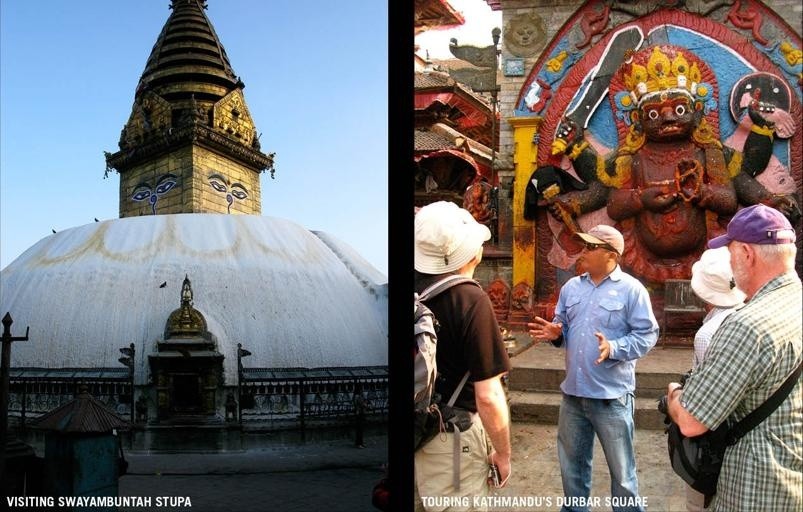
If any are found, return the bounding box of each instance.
[488,467,500,488]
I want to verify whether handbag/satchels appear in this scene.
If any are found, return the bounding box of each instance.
[666,404,734,509]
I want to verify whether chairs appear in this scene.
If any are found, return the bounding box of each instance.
[486,280,509,329]
[508,282,536,331]
[662,279,707,350]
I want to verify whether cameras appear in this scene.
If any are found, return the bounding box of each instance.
[658,372,689,426]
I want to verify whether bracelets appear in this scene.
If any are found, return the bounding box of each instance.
[672,386,683,396]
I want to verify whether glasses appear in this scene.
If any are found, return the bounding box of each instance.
[583,241,614,253]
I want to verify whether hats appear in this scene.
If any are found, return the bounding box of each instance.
[573,223,626,256]
[691,243,749,308]
[413,197,492,274]
[707,202,797,250]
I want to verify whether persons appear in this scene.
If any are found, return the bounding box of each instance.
[666,203,801,511]
[527,224,658,512]
[413,199,514,512]
[689,245,747,375]
[602,43,737,282]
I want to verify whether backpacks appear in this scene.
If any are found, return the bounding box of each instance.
[414,273,488,455]
